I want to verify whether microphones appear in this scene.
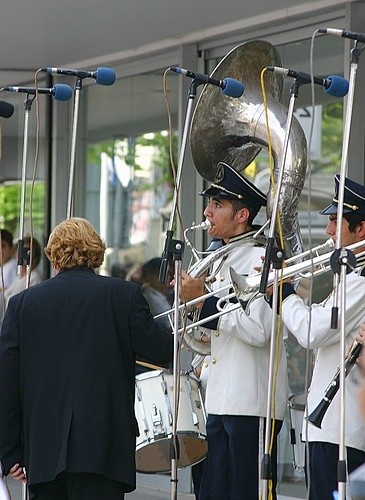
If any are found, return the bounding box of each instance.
[0,67,116,118]
[170,67,245,98]
[267,66,349,97]
[318,28,365,43]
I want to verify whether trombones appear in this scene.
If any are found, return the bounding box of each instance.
[153,237,365,337]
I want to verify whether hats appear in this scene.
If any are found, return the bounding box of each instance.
[319,175,365,215]
[198,162,269,207]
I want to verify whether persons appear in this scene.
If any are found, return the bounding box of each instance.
[0,217,179,500]
[1,164,365,500]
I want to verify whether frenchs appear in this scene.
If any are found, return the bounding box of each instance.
[169,39,309,357]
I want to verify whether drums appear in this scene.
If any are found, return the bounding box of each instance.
[133,370,210,474]
[288,391,311,469]
